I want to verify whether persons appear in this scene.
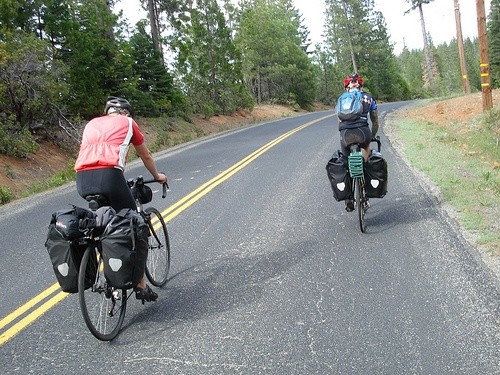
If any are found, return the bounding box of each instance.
[74,95,167,301]
[336,73,379,211]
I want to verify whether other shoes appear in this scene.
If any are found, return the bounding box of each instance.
[345,202,354,212]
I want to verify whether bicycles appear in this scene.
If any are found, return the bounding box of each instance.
[341,136,381,230]
[76,169,171,342]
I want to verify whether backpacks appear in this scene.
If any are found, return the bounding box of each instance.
[337,91,363,121]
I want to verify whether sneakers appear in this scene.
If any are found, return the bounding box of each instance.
[135,284,158,300]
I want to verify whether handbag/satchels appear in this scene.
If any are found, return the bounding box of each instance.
[101,208,151,290]
[45,206,96,294]
[365,150,387,199]
[326,150,353,201]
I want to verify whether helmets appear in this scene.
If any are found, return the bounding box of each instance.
[104,96,134,118]
[344,72,363,88]
